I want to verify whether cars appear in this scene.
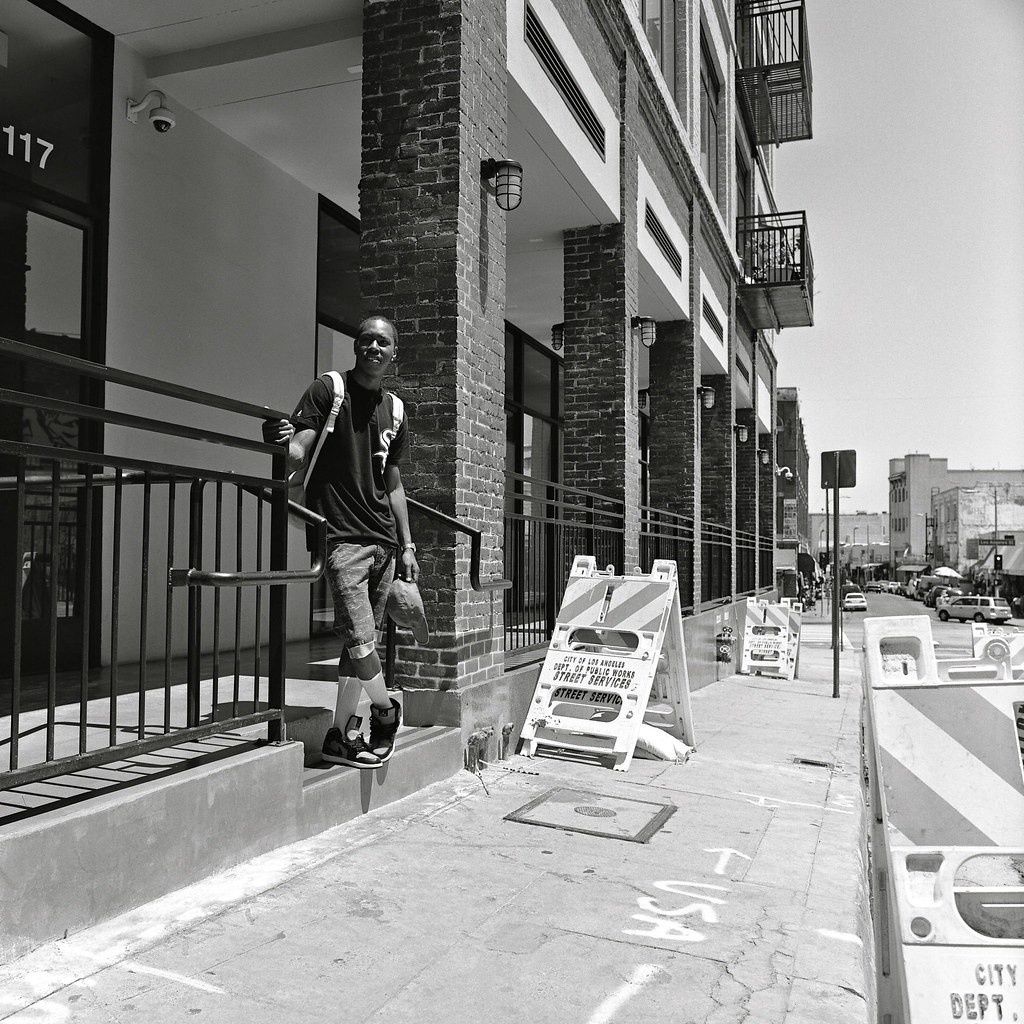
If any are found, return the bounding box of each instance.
[864,582,881,593]
[842,592,867,611]
[878,576,963,607]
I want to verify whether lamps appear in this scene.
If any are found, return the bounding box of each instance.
[733,423,748,442]
[550,322,565,351]
[631,316,657,349]
[638,386,650,408]
[482,155,523,211]
[756,449,769,464]
[697,385,716,409]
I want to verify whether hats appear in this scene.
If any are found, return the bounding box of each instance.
[385,578,429,646]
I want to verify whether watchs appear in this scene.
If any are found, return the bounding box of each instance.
[401,543,416,554]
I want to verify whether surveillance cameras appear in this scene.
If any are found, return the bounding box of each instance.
[149,107,176,133]
[785,472,792,481]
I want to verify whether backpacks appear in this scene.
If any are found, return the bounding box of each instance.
[285,372,405,529]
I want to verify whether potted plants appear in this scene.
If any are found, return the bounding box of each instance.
[746,233,801,282]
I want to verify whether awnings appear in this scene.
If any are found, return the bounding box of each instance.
[982,545,1024,575]
[797,552,815,572]
[896,565,928,572]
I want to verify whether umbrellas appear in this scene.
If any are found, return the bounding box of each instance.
[935,567,964,579]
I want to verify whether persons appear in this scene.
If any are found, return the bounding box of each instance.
[262,316,420,769]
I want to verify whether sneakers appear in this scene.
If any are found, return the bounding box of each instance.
[322,715,383,768]
[369,696,401,763]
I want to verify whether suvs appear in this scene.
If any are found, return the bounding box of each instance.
[841,584,861,599]
[937,596,1013,623]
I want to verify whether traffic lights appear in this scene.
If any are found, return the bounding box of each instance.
[995,555,1003,570]
